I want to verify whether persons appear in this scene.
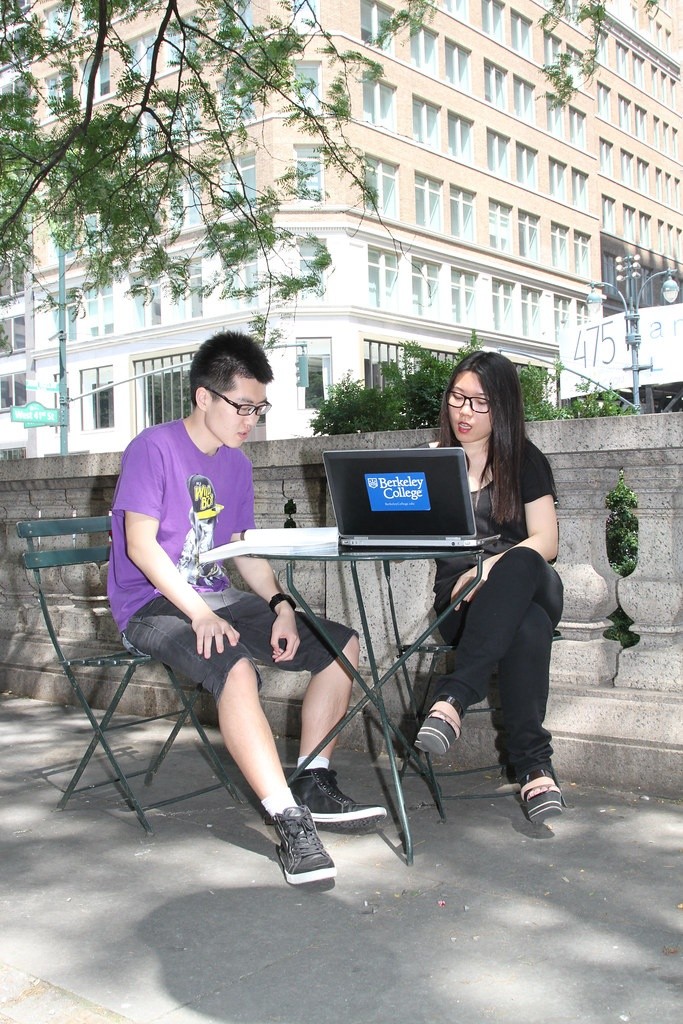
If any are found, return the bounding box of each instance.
[414,352,567,824]
[106,332,387,885]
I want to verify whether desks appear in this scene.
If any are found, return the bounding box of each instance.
[246,544,485,867]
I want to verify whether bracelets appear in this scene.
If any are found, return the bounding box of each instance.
[269,593,296,612]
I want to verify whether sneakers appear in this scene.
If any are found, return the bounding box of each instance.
[274,805,337,885]
[292,767,388,829]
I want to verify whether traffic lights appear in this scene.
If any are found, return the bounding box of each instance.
[296,355,309,388]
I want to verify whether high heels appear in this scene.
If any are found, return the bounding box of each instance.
[413,695,463,756]
[519,769,563,823]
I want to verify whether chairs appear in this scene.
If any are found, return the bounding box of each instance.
[15,517,243,837]
[383,559,563,825]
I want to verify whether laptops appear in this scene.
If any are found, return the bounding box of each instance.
[323,446,501,549]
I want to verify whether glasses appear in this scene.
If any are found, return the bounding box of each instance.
[446,390,491,414]
[205,385,272,416]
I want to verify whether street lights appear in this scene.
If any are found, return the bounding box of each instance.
[588,255,680,413]
